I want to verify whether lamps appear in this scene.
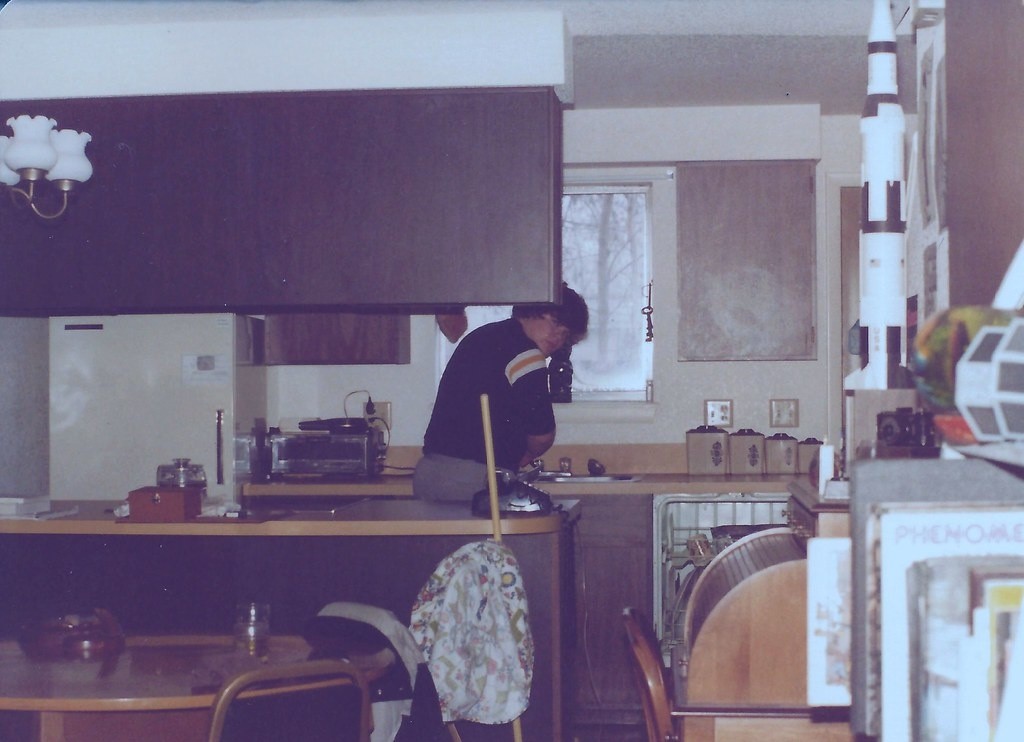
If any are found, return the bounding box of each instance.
[0,114,93,220]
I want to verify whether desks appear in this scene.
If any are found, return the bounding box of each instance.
[0,633,393,742]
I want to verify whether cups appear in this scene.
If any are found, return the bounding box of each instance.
[685,532,714,566]
[232,600,272,665]
[157,457,207,501]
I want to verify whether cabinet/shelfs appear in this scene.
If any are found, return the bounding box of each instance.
[247,312,412,365]
[551,493,654,725]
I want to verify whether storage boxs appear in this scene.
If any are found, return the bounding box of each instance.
[128,486,201,522]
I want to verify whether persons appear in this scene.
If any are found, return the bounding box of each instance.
[412,283,588,503]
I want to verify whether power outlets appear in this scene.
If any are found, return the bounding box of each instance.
[362,401,392,431]
[769,398,800,428]
[704,400,733,427]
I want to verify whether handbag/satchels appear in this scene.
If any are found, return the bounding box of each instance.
[305,601,452,742]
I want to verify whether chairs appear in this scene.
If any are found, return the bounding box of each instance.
[383,540,535,742]
[206,659,370,742]
[623,605,675,742]
